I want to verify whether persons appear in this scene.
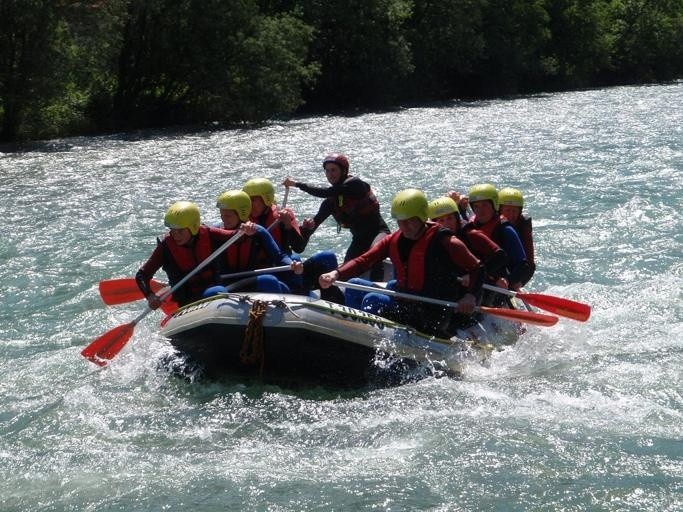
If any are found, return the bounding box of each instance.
[135,178,338,311]
[284,154,391,281]
[318,183,537,337]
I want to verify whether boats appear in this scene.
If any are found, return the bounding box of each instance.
[158,257,533,395]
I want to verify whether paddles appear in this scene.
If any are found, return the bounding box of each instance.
[80,229,246,367]
[331,280,558,326]
[456,276,590,322]
[98,261,296,305]
[159,218,283,315]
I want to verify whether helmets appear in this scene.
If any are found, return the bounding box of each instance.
[467,184,499,212]
[163,200,199,236]
[322,153,348,172]
[498,188,523,216]
[429,197,460,226]
[390,189,428,224]
[215,189,251,222]
[243,178,274,207]
